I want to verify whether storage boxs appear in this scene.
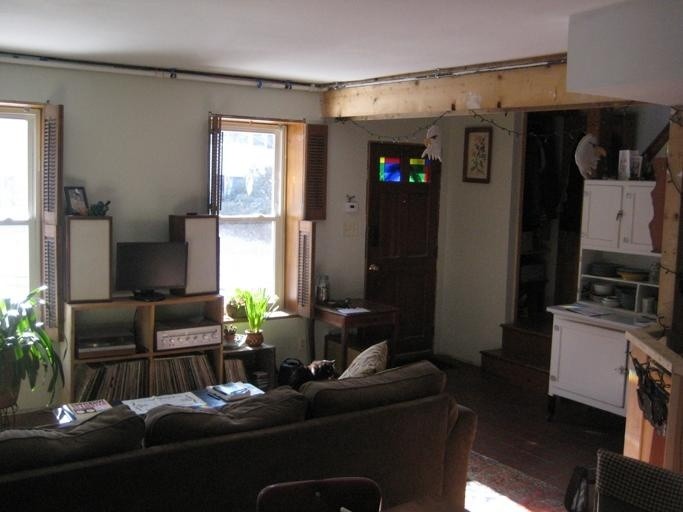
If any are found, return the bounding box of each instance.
[522,232,533,250]
[520,264,544,280]
[326,335,340,363]
[347,336,432,369]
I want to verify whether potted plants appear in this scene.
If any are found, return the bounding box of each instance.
[0,286,68,408]
[245,289,279,346]
[224,325,237,343]
[226,289,247,318]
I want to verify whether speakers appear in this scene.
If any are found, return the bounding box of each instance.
[64,214,112,304]
[169,213,219,297]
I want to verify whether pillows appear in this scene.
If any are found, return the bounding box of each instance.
[142,385,310,449]
[593,450,683,512]
[338,341,388,380]
[298,360,447,418]
[1,404,145,475]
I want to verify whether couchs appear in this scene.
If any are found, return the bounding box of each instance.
[0,360,478,512]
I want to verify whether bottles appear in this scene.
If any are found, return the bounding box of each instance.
[318,275,328,288]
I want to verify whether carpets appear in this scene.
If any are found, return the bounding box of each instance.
[384,451,567,512]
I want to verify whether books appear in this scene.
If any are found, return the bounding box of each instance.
[223,359,270,390]
[74,355,216,404]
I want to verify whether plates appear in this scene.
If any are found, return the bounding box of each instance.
[589,261,659,313]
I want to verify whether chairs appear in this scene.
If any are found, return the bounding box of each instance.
[564,466,596,512]
[256,476,383,512]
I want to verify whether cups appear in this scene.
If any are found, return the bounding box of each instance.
[317,287,328,305]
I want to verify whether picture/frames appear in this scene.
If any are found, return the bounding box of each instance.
[64,186,88,215]
[463,125,493,184]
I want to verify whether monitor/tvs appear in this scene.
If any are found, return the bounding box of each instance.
[115,241,189,301]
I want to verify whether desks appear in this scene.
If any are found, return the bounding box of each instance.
[308,299,399,372]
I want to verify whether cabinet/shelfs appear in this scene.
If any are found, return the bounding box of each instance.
[520,116,564,305]
[224,343,276,390]
[60,295,223,404]
[577,180,661,317]
[547,314,627,417]
